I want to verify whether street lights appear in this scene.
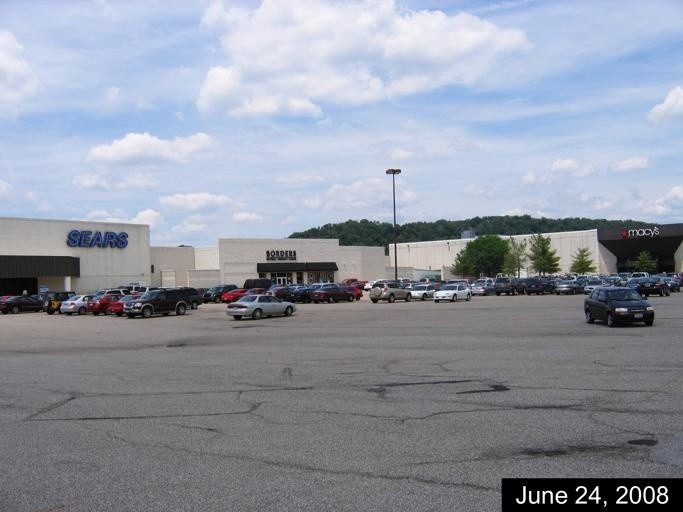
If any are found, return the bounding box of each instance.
[385,167,402,281]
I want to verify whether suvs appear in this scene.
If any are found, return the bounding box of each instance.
[177,286,203,311]
[40,289,77,315]
[123,286,186,318]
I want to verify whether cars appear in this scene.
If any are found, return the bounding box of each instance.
[57,293,93,316]
[207,279,472,303]
[107,294,141,316]
[580,286,654,327]
[85,293,123,315]
[473,271,683,297]
[225,293,297,320]
[0,289,41,315]
